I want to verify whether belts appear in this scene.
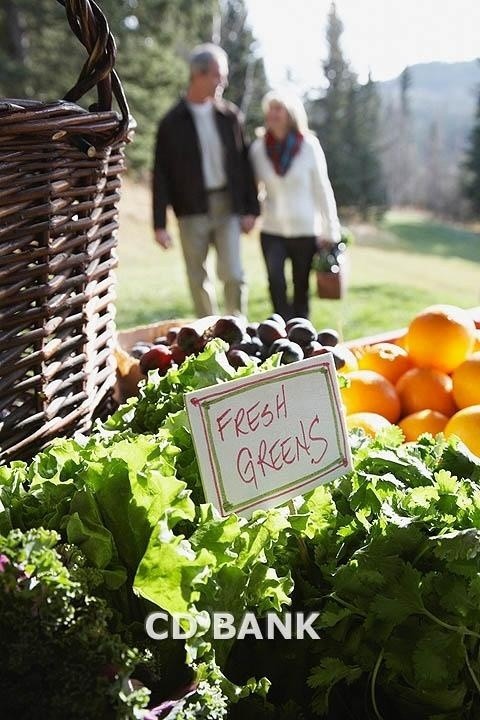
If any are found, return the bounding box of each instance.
[205,185,229,195]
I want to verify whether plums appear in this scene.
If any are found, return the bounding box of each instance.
[130,313,344,394]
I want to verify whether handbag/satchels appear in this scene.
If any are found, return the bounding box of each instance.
[316,247,347,300]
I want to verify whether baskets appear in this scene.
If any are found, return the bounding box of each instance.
[0,0,139,465]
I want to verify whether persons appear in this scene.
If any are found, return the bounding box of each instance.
[150,42,260,318]
[250,88,354,320]
